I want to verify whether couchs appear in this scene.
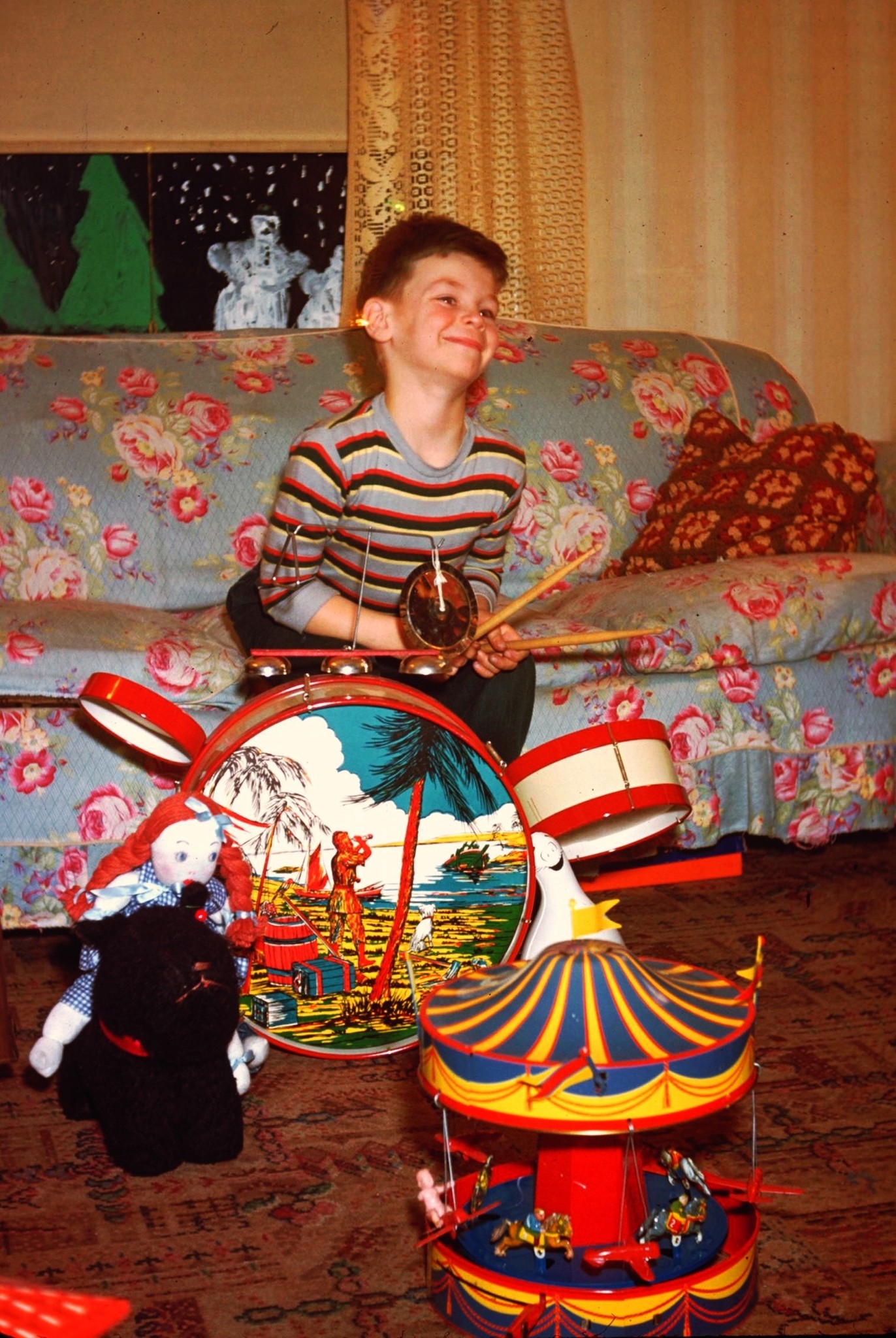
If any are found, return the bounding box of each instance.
[1,312,896,927]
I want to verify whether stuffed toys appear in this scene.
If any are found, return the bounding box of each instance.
[55,880,246,1177]
[28,790,267,1093]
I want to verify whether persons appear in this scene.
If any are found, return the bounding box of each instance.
[219,210,537,767]
[523,1207,546,1235]
[415,1167,457,1226]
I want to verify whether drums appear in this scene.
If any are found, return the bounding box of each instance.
[180,675,534,1060]
[79,672,206,766]
[504,719,692,863]
